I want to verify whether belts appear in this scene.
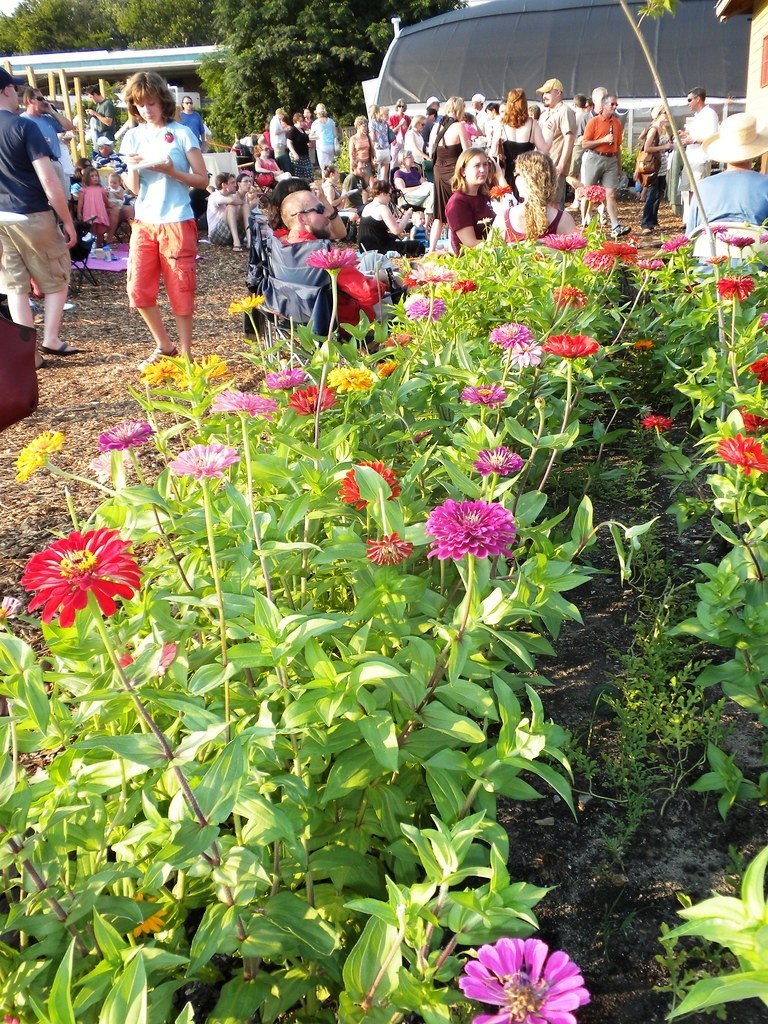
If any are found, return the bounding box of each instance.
[586,148,617,157]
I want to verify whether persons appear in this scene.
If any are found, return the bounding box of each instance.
[0,67,768,369]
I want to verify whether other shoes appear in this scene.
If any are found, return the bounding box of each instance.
[41,343,92,355]
[565,203,661,238]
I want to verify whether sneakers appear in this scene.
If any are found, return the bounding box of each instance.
[139,347,178,369]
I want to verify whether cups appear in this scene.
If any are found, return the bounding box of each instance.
[248,193,255,205]
[362,250,379,272]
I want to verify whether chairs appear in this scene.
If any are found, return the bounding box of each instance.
[59,215,98,297]
[685,218,768,260]
[242,165,424,372]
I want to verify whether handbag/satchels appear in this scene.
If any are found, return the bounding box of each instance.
[387,124,396,143]
[633,128,660,188]
[334,123,341,155]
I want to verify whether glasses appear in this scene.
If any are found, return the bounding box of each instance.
[262,148,268,151]
[605,103,618,107]
[688,96,698,102]
[399,106,405,108]
[33,96,45,101]
[183,102,192,104]
[1,85,17,93]
[419,122,425,126]
[291,203,324,216]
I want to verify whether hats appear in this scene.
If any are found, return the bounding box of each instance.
[97,136,113,146]
[0,67,25,88]
[701,113,768,162]
[651,105,665,119]
[276,109,287,115]
[426,96,441,109]
[536,79,563,94]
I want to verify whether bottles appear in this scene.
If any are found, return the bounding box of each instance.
[103,245,111,262]
[385,267,405,305]
[607,125,614,146]
[387,251,401,259]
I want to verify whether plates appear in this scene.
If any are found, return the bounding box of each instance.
[128,158,166,169]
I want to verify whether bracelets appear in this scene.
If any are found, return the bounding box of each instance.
[694,139,696,144]
[328,206,339,220]
[50,110,57,117]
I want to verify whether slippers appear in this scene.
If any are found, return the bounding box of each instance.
[233,246,244,252]
